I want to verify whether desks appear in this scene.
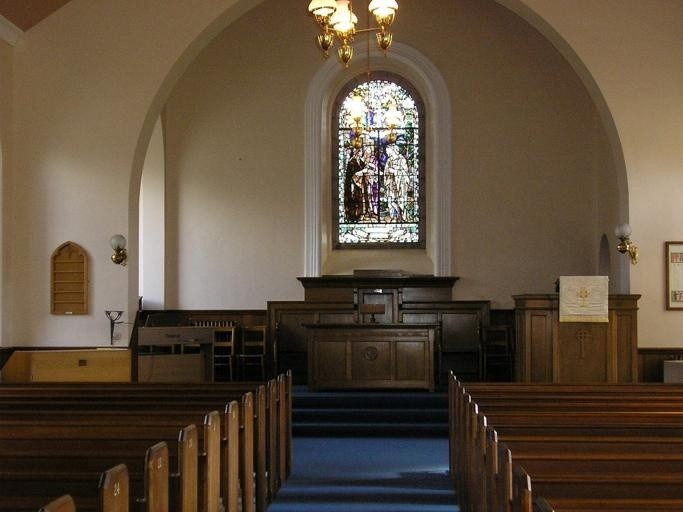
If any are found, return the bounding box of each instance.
[136,325,213,382]
[301,321,441,391]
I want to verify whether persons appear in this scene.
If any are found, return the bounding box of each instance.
[383,144,410,222]
[344,147,370,222]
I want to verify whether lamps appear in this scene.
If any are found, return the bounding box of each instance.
[614,222,638,266]
[305,1,400,70]
[108,233,127,265]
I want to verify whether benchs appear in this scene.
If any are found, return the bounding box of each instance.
[446,368,683,512]
[0,369,294,511]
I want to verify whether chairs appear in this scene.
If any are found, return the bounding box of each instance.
[212,324,268,379]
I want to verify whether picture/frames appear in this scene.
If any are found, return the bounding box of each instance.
[665,240,683,312]
